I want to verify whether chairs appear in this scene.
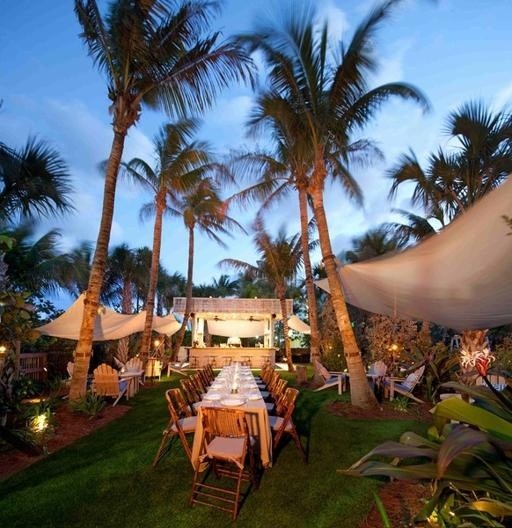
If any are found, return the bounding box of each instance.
[147,362,313,521]
[313,359,427,409]
[61,355,167,407]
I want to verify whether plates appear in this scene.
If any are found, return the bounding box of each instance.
[204,364,264,407]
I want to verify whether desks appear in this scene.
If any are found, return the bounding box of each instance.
[188,347,277,368]
